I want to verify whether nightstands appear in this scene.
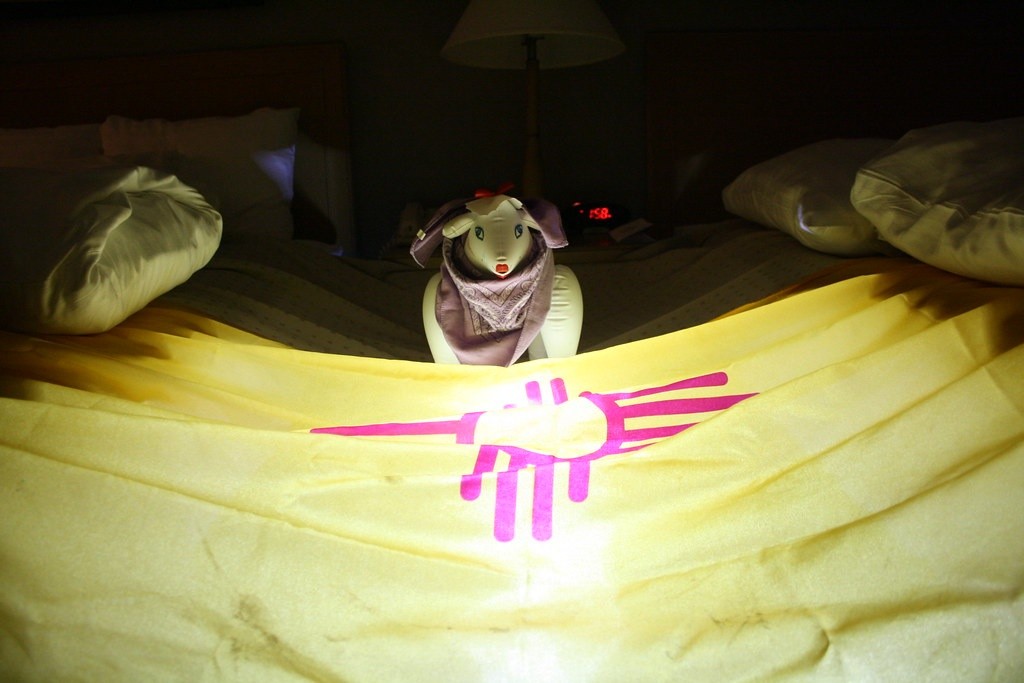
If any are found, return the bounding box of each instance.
[380,227,657,273]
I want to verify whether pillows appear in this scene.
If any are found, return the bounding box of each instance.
[99,106,302,246]
[720,138,910,258]
[0,157,224,337]
[850,118,1024,286]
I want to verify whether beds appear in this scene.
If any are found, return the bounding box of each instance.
[645,25,1024,682]
[1,40,357,682]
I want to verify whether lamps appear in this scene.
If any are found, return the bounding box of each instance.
[437,1,627,212]
